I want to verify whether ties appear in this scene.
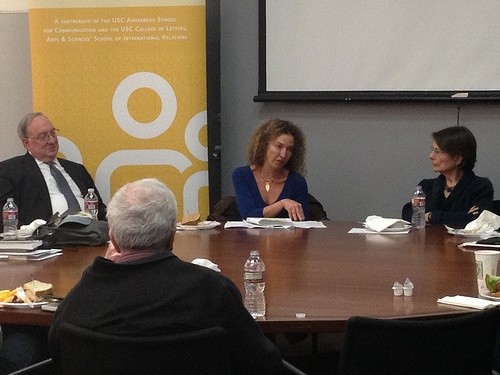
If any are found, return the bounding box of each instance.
[46,161,82,211]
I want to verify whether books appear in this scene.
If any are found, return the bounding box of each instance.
[247,217,293,226]
[0,240,63,261]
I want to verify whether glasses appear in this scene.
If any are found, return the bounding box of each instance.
[24,128,60,140]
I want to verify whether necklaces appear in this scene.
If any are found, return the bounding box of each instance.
[446,185,453,192]
[261,173,273,192]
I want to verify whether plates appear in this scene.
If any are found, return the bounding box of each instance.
[448,230,500,237]
[0,299,59,308]
[0,230,32,240]
[362,225,412,232]
[176,221,221,229]
[481,292,500,300]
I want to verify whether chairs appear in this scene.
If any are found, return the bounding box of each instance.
[51,321,232,375]
[335,307,500,375]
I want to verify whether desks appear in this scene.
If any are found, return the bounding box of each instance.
[0,218,500,375]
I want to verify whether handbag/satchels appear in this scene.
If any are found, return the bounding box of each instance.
[26,212,110,246]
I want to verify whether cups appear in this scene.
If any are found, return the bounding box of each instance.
[473,250,500,293]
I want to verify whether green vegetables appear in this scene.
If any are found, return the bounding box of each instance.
[485,274,500,293]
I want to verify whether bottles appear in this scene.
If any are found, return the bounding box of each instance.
[83,189,98,220]
[243,250,267,318]
[411,185,425,229]
[3,197,18,241]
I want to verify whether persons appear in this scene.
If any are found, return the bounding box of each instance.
[0,112,107,234]
[48,178,283,375]
[232,118,327,221]
[402,126,494,230]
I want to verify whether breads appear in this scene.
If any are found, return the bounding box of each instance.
[494,282,500,297]
[24,279,53,303]
